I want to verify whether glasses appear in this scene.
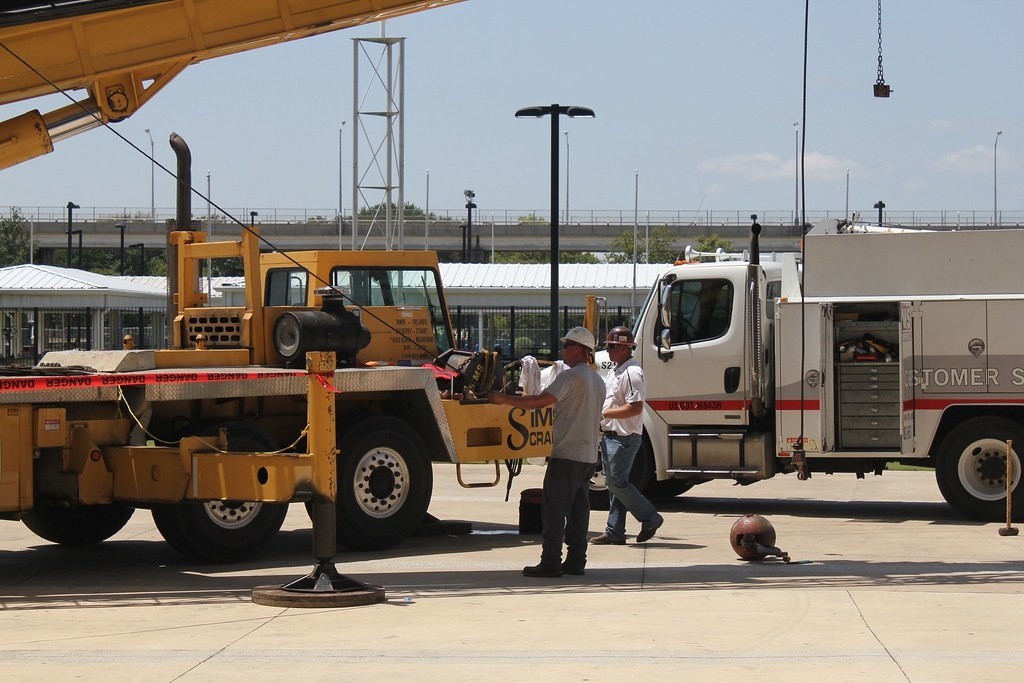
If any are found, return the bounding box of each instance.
[564,343,577,349]
[608,344,622,350]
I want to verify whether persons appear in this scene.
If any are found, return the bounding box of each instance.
[486,327,607,578]
[589,326,663,545]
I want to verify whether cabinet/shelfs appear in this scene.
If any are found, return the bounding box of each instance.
[836,323,899,449]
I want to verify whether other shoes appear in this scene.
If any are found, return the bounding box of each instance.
[562,557,586,574]
[523,556,563,577]
[589,535,626,544]
[636,517,663,542]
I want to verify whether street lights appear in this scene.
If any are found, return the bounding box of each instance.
[129,242,146,349]
[458,225,468,263]
[65,228,83,352]
[491,212,495,263]
[514,104,597,363]
[563,131,569,226]
[630,168,640,331]
[846,168,851,221]
[465,201,477,263]
[873,200,886,227]
[425,169,430,249]
[993,130,1003,228]
[145,129,154,218]
[207,171,211,307]
[66,200,82,348]
[793,122,799,226]
[338,120,346,222]
[114,223,125,276]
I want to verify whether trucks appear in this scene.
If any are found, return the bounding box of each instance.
[586,218,1024,524]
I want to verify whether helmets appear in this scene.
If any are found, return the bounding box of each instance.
[603,326,638,346]
[560,327,595,350]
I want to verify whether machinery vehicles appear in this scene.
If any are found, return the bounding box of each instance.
[0,0,610,610]
